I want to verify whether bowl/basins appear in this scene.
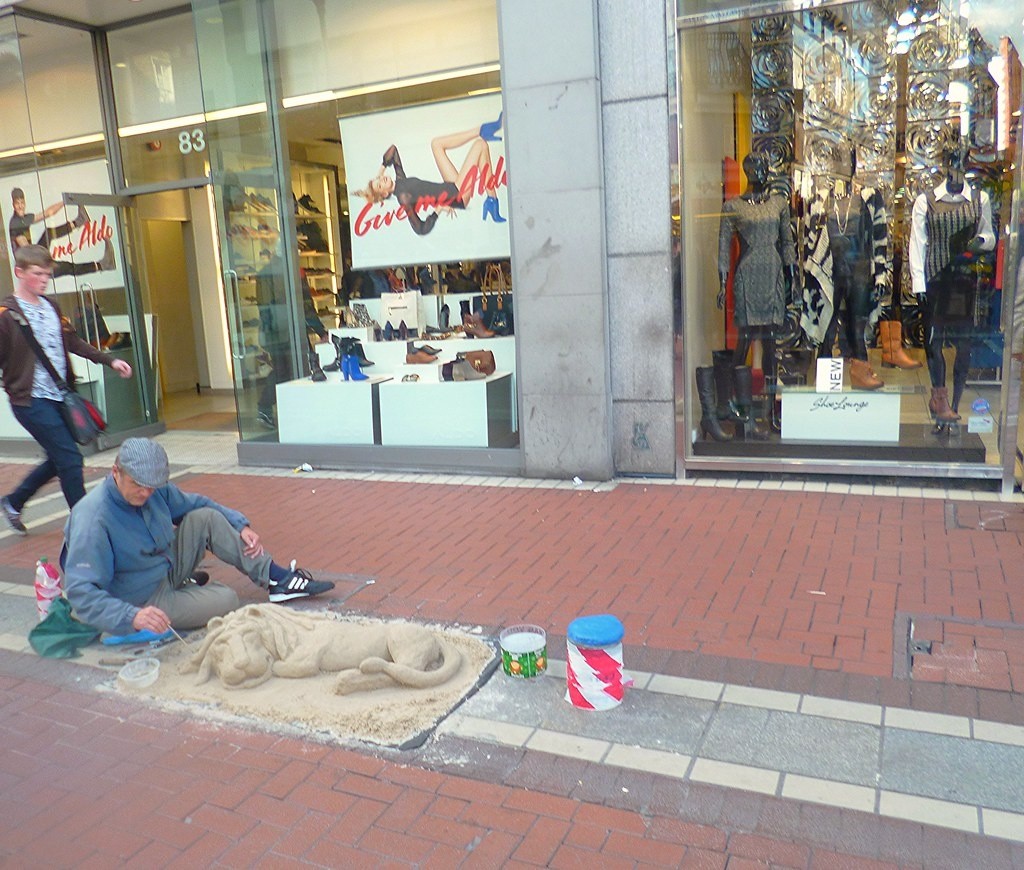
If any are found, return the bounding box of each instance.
[119,657,161,688]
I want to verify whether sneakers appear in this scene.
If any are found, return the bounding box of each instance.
[179,569,209,590]
[269,558,335,603]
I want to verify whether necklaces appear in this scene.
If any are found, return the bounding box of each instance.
[833,195,853,235]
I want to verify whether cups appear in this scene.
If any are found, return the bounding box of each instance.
[499,624,548,681]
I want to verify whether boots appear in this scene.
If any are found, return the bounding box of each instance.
[72,204,90,228]
[97,239,117,271]
[927,386,960,422]
[879,320,923,370]
[696,348,782,442]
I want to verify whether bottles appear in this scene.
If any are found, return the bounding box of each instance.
[34,557,64,624]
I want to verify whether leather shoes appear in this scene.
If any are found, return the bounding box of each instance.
[100,332,130,352]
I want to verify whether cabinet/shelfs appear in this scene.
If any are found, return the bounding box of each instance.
[275,292,519,448]
[194,149,344,390]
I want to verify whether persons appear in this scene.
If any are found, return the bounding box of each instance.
[58,439,335,636]
[257,238,328,431]
[801,179,887,362]
[350,111,506,235]
[9,188,117,278]
[445,263,466,293]
[0,244,131,536]
[909,148,996,433]
[342,268,392,299]
[717,153,796,432]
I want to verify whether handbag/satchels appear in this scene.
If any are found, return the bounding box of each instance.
[456,349,495,375]
[27,596,103,659]
[59,390,104,444]
[471,264,514,335]
[74,305,109,341]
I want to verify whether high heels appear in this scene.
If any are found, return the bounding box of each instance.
[483,196,507,223]
[308,263,496,382]
[848,358,885,389]
[480,112,504,141]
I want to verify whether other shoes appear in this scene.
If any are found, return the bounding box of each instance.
[221,172,335,361]
[256,411,277,430]
[0,495,26,535]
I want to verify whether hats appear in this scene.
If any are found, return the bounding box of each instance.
[118,437,169,489]
